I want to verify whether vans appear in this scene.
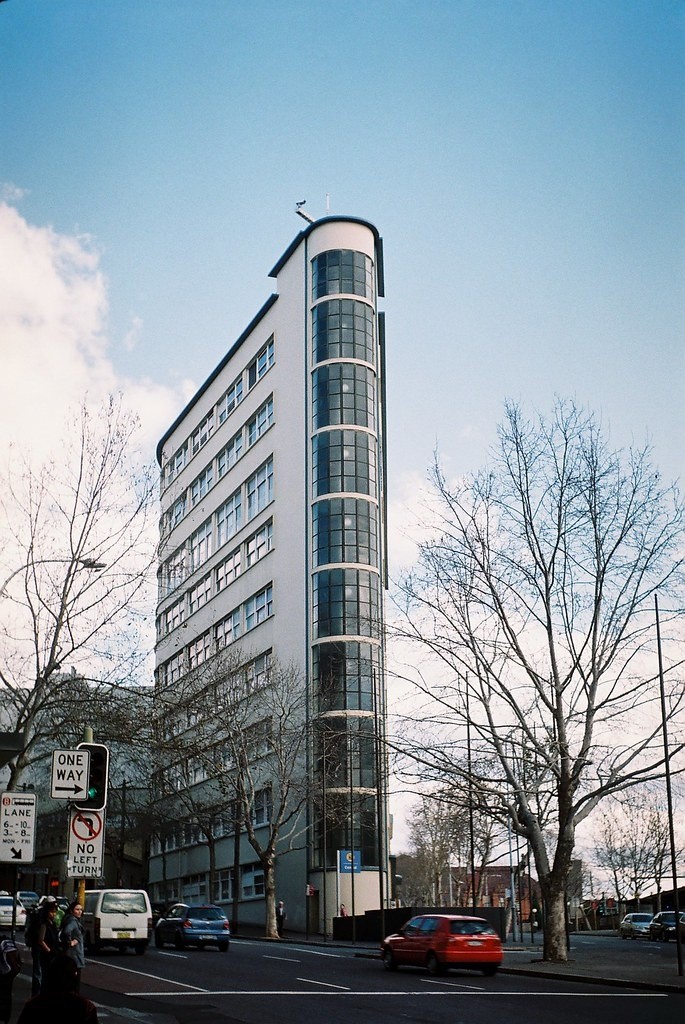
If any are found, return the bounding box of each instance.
[74,890,153,955]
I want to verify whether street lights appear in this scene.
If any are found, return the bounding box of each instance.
[433,685,492,907]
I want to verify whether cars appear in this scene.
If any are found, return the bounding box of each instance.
[15,891,40,909]
[155,903,231,952]
[0,895,28,929]
[620,913,654,939]
[650,912,685,942]
[381,913,504,978]
[32,894,71,913]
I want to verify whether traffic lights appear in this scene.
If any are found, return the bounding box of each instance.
[74,743,109,813]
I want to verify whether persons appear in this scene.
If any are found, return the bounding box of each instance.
[339,904,349,917]
[0,895,98,1023]
[275,901,287,939]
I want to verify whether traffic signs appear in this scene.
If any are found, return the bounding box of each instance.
[51,751,86,801]
